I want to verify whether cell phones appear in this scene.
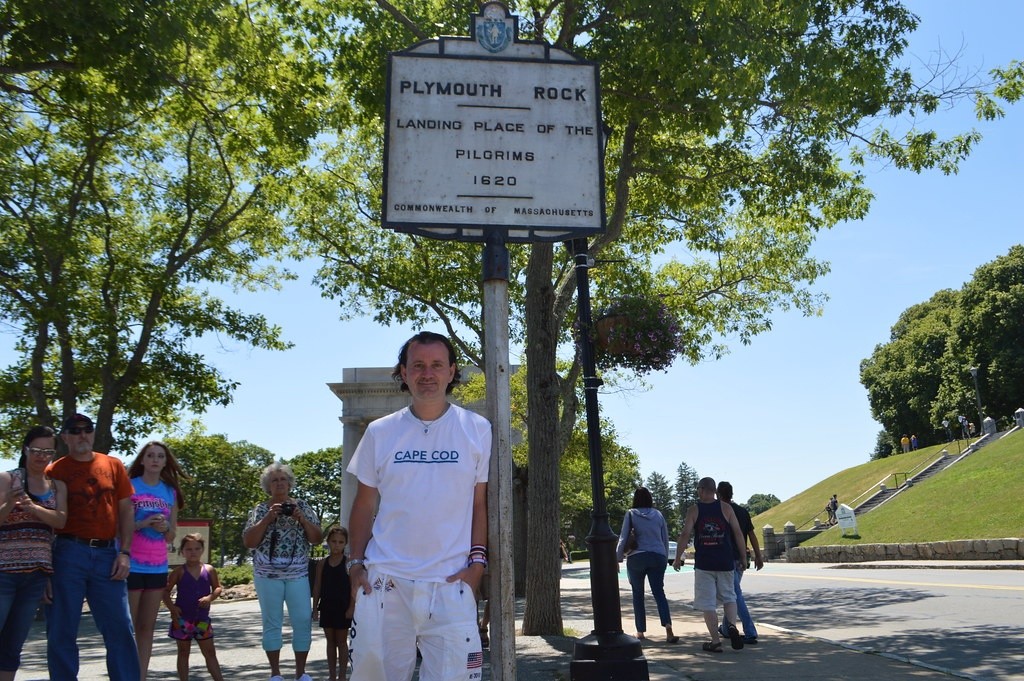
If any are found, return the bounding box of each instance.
[11,468,25,490]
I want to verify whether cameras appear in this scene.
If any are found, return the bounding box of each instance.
[280,503,296,516]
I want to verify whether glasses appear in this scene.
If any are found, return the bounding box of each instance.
[26,447,56,458]
[64,426,93,434]
[697,487,701,489]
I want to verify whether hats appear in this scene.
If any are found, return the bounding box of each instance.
[64,413,93,427]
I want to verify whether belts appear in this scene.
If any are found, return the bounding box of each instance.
[58,534,115,548]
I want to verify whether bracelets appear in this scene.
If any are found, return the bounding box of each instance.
[161,527,169,534]
[468,544,487,568]
[120,550,130,556]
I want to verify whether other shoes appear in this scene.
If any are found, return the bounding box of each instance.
[270,674,284,681]
[742,635,758,644]
[294,674,313,681]
[718,625,729,638]
[637,636,645,640]
[666,635,679,642]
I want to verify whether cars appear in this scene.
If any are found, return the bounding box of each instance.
[667,541,686,566]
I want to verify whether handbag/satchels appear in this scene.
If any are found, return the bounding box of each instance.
[623,509,638,556]
[826,507,831,511]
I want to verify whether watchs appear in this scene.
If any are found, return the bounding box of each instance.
[346,559,363,576]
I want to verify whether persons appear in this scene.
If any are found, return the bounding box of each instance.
[910,435,918,451]
[116,442,189,681]
[617,487,679,644]
[672,477,748,652]
[163,534,224,681]
[713,481,764,643]
[942,418,955,442]
[347,332,493,681]
[243,464,323,681]
[312,527,355,681]
[901,434,909,453]
[42,413,141,681]
[828,495,838,526]
[0,426,68,681]
[958,413,976,439]
[565,536,575,563]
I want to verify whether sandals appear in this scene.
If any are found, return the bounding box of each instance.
[703,642,723,653]
[728,624,743,650]
[479,622,489,647]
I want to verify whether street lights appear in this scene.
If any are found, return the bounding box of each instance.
[970,365,986,435]
